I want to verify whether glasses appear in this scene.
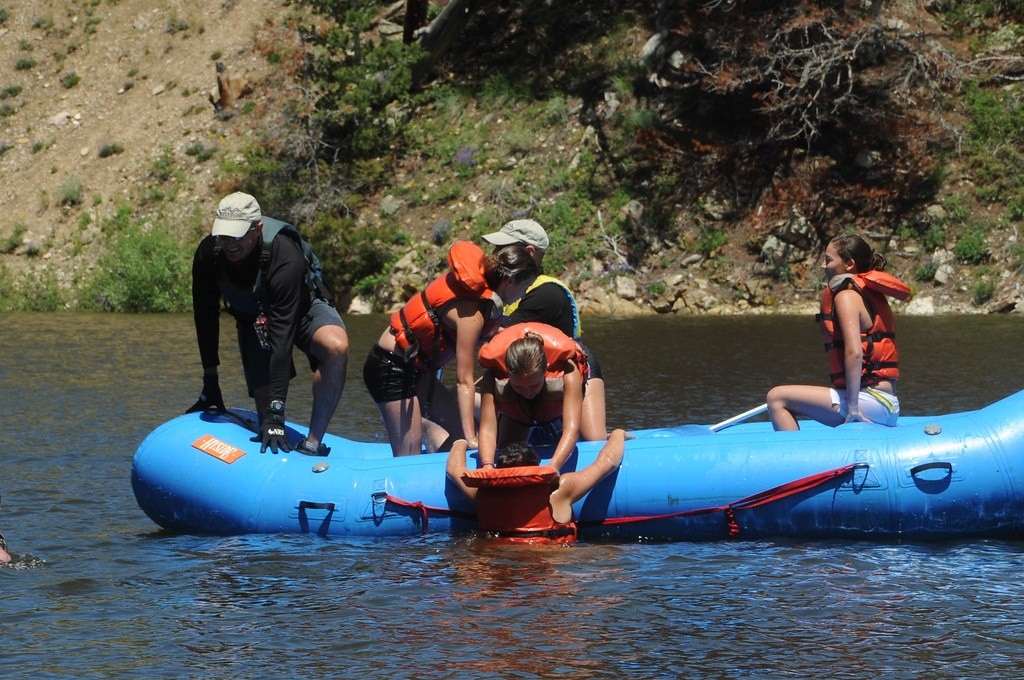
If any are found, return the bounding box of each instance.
[217,226,257,240]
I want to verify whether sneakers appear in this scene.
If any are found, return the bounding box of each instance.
[295,434,331,456]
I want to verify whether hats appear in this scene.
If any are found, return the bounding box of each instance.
[211,191,261,239]
[481,218,549,254]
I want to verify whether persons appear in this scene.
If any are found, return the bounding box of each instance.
[478,321,608,476]
[182,191,348,456]
[766,234,901,430]
[445,219,582,420]
[362,243,538,459]
[445,426,637,546]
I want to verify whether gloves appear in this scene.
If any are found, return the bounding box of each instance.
[250,397,293,454]
[186,371,228,414]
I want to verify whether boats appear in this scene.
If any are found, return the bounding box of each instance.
[127,385,1024,537]
